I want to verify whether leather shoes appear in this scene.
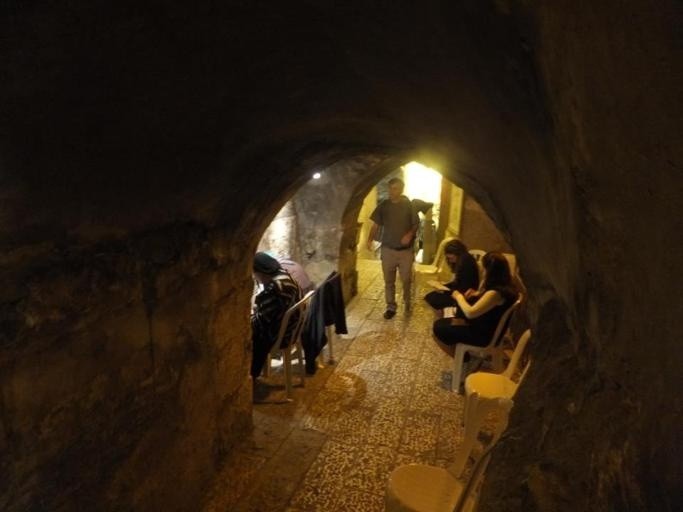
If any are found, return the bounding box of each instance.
[384,308,397,319]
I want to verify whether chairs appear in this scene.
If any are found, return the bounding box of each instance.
[410,238,530,479]
[263,260,346,399]
[383,398,513,512]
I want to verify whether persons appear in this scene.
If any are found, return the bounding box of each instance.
[423,240,515,384]
[366,176,418,320]
[251,251,317,382]
